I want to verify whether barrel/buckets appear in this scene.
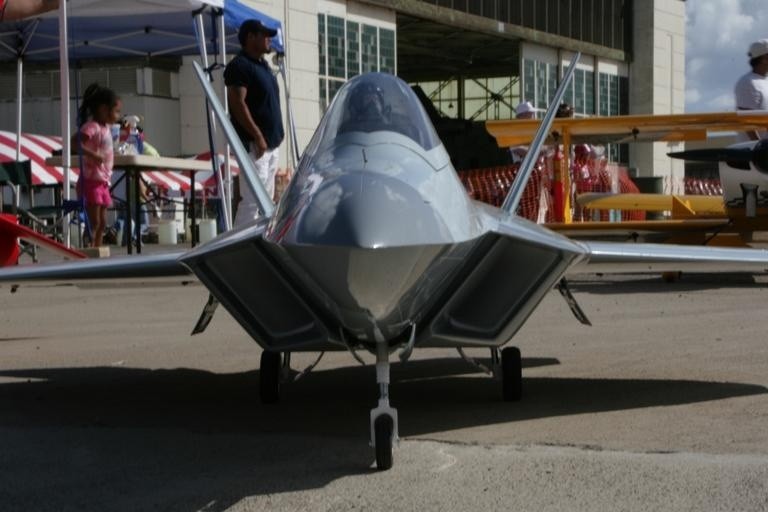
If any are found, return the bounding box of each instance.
[198,218,217,242]
[158,220,178,245]
[633,177,663,217]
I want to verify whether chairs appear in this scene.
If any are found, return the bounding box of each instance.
[1,158,37,264]
[24,180,62,243]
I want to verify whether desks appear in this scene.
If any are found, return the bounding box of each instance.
[49,154,214,255]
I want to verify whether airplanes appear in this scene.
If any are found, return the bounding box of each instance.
[0,210,88,269]
[0,52,768,472]
[484,110,768,279]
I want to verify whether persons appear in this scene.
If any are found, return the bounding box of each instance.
[510,102,547,164]
[132,174,156,248]
[223,19,286,230]
[735,38,768,141]
[71,82,123,248]
[137,124,160,158]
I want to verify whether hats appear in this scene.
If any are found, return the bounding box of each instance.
[237,18,278,37]
[514,101,540,117]
[746,38,768,60]
